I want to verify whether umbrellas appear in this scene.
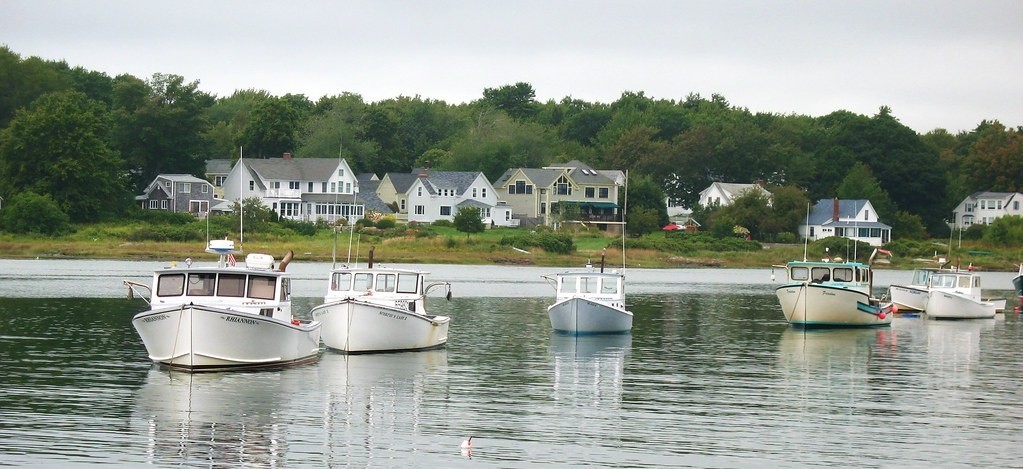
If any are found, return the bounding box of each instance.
[663,224,679,231]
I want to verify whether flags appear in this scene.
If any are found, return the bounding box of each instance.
[228,255,236,266]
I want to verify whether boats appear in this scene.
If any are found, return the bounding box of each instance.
[770,200,894,328]
[538,208,634,336]
[311,187,454,354]
[889,213,1008,312]
[122,146,323,373]
[925,222,996,318]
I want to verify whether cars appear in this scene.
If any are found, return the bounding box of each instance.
[663,224,680,231]
[673,220,687,230]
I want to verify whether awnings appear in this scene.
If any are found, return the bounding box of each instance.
[559,201,590,208]
[589,202,621,209]
